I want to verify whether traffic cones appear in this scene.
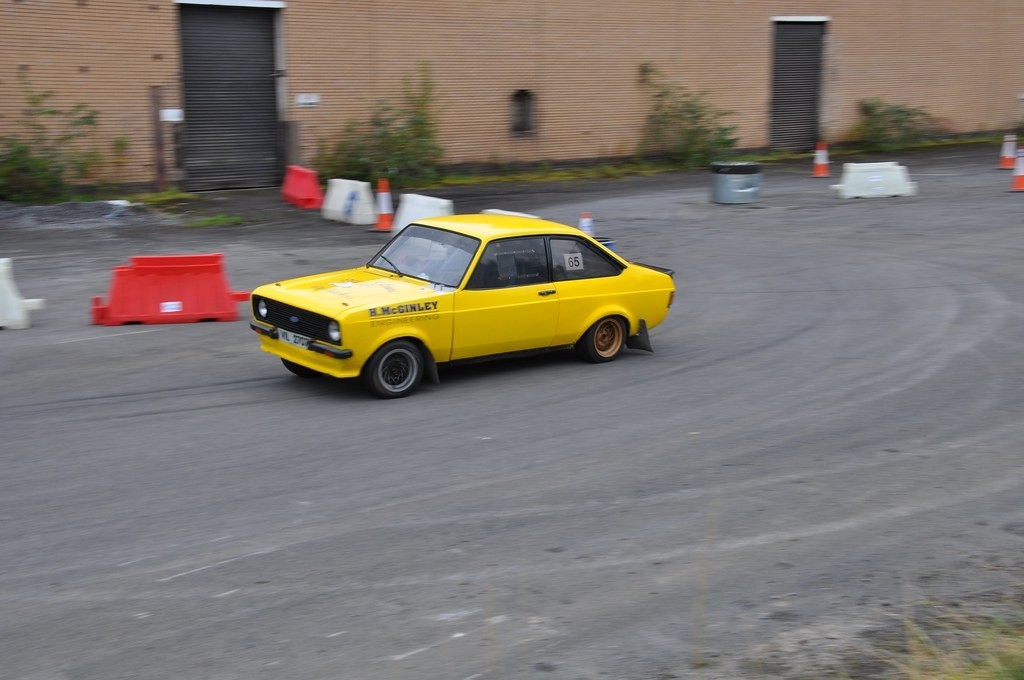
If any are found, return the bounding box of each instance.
[993,133,1016,169]
[1006,146,1024,192]
[366,178,395,232]
[810,139,832,178]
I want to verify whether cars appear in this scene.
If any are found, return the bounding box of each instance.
[249,213,675,398]
